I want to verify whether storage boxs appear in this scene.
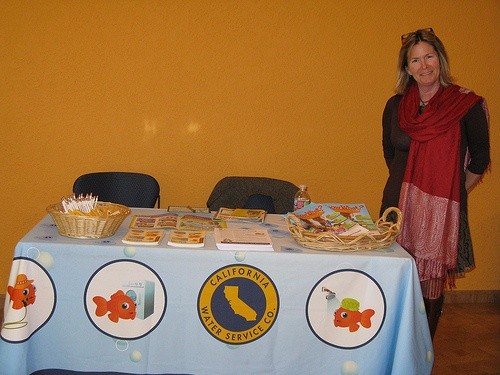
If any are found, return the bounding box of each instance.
[45,198,131,241]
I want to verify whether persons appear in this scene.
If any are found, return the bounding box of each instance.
[381,27,491,341]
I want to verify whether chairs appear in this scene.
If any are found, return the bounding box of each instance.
[72,172,160,209]
[207,176,300,215]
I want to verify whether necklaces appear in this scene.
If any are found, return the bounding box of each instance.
[419,97,430,113]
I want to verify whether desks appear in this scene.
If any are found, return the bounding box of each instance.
[17,198,415,374]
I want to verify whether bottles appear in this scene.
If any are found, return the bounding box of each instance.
[294,185,311,212]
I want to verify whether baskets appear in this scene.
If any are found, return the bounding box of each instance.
[46,201,131,240]
[286,207,402,252]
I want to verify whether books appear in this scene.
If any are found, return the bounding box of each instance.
[129,213,229,232]
[121,227,166,245]
[212,207,267,224]
[167,229,207,248]
[214,226,275,251]
[282,202,381,238]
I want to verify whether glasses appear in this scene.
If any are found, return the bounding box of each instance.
[401,28,435,45]
